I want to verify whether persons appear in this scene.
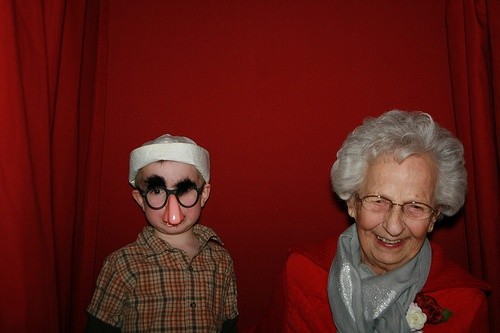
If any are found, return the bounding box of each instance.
[261,111,495,333]
[86,133,239,333]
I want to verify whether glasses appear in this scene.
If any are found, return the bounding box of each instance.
[354,193,437,219]
[137,180,206,210]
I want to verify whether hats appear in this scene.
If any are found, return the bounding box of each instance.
[128,134,210,187]
